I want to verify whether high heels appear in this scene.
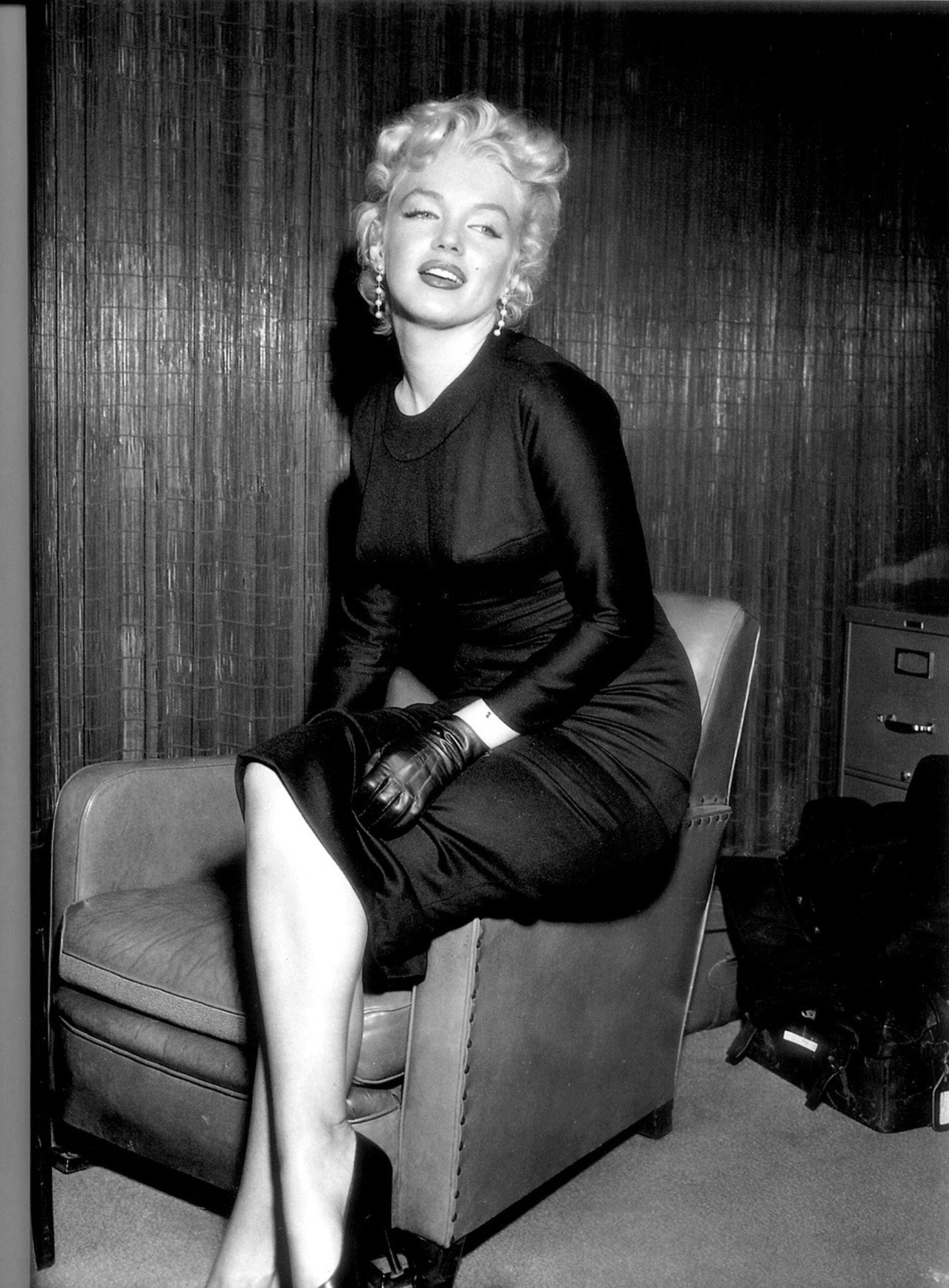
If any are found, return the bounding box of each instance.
[314,1131,404,1288]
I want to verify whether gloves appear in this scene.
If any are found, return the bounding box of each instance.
[347,714,492,840]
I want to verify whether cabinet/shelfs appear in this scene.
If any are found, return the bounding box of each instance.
[832,597,949,809]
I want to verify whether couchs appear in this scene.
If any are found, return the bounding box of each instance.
[44,588,767,1287]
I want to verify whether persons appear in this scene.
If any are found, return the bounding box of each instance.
[205,97,701,1287]
